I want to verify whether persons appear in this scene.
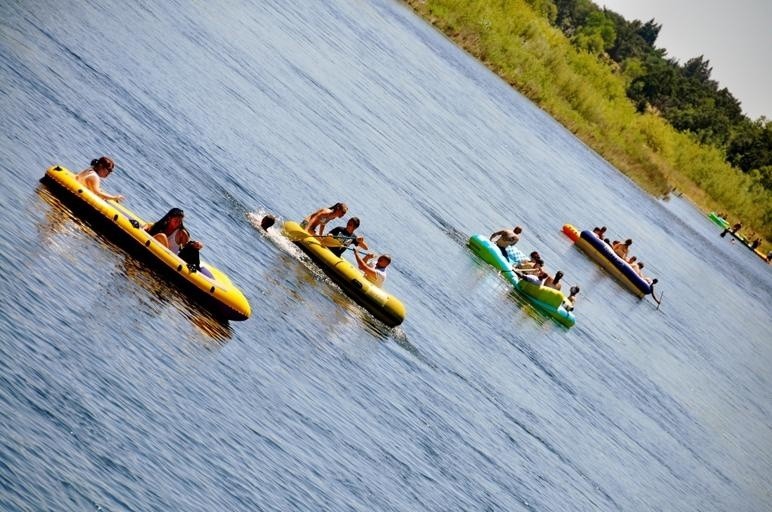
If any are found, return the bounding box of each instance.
[146,207,203,256]
[515,252,580,311]
[353,247,391,287]
[301,202,348,237]
[718,211,772,265]
[261,215,275,231]
[327,217,368,259]
[592,226,660,305]
[77,156,125,203]
[489,225,522,262]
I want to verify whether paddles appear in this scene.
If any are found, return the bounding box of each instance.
[288,231,357,241]
[500,270,539,273]
[320,236,376,258]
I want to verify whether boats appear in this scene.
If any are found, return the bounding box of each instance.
[280,220,406,327]
[467,233,581,329]
[575,230,653,300]
[44,164,253,323]
[562,223,580,241]
[710,211,731,229]
